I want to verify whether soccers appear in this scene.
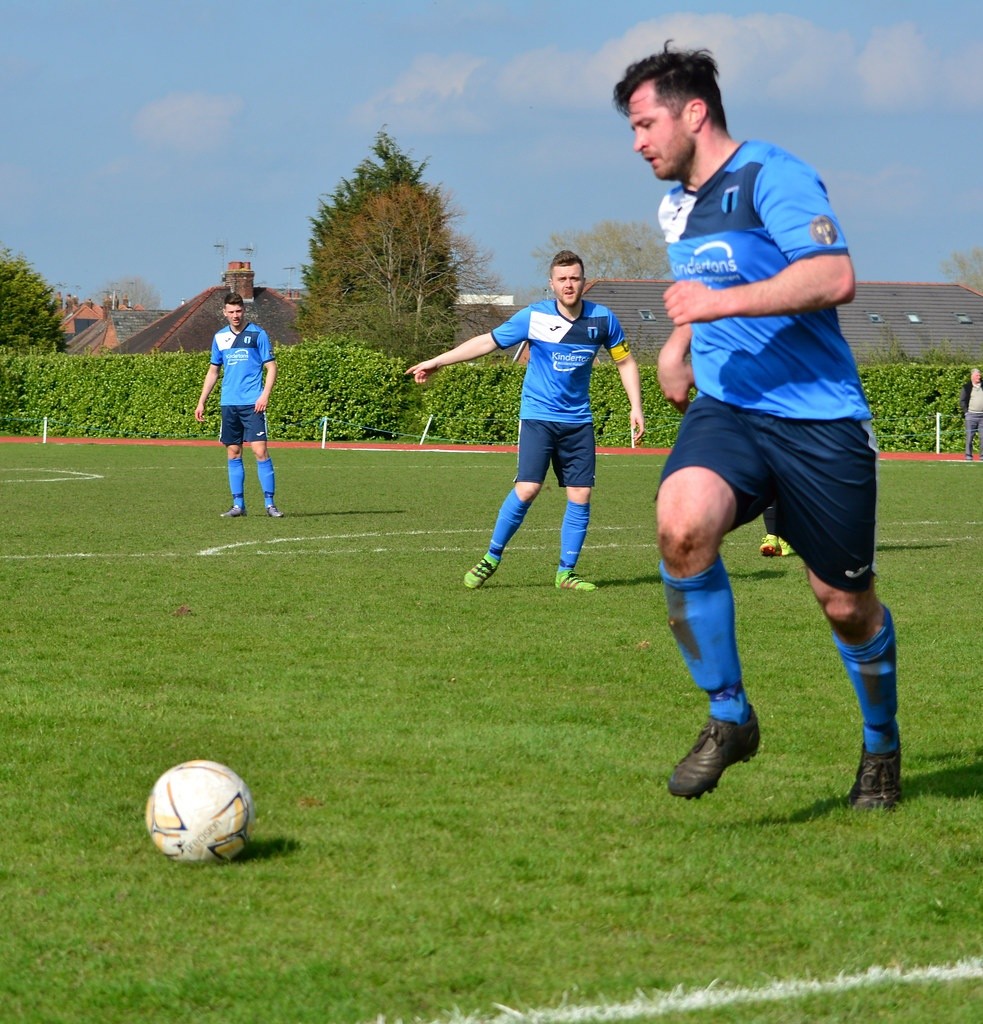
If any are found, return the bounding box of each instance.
[143,760,256,866]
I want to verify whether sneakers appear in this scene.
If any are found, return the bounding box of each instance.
[219,505,248,518]
[758,536,782,557]
[463,553,500,589]
[265,505,284,517]
[554,569,598,591]
[847,736,902,814]
[776,537,798,556]
[666,703,761,799]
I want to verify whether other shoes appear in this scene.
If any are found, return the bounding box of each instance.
[979,456,983,460]
[966,454,973,460]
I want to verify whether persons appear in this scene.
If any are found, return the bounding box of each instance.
[614,47,902,812]
[960,368,983,461]
[407,251,646,591]
[195,293,285,517]
[759,503,795,556]
